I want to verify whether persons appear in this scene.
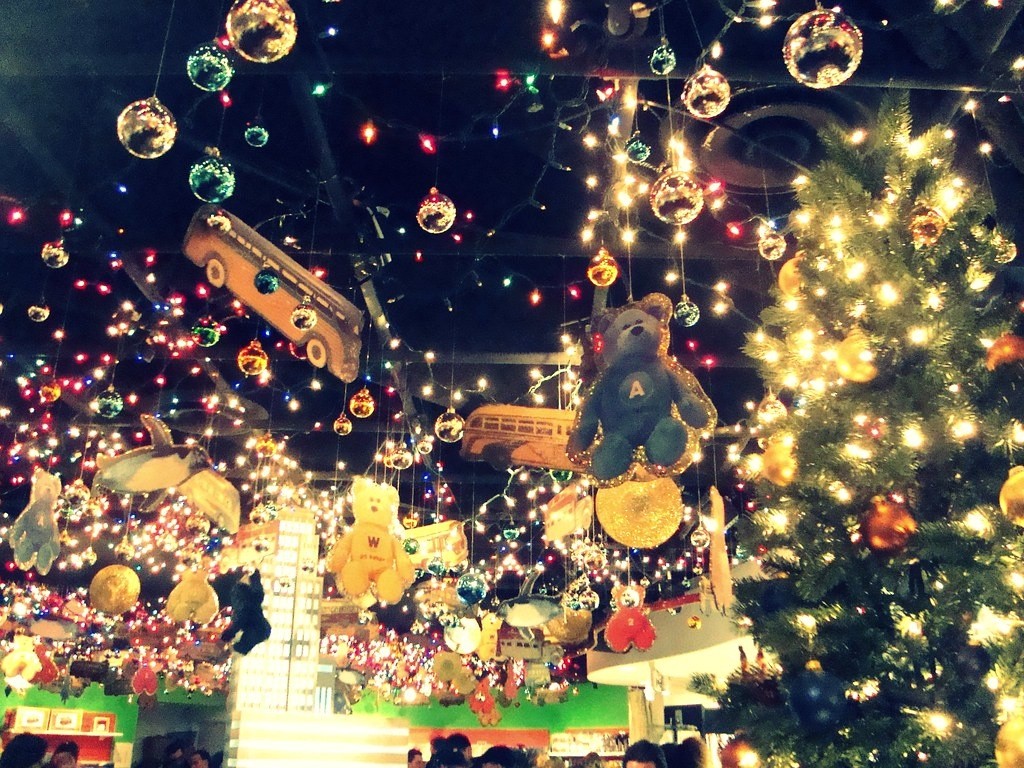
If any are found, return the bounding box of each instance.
[407,734,722,768]
[0,734,210,768]
